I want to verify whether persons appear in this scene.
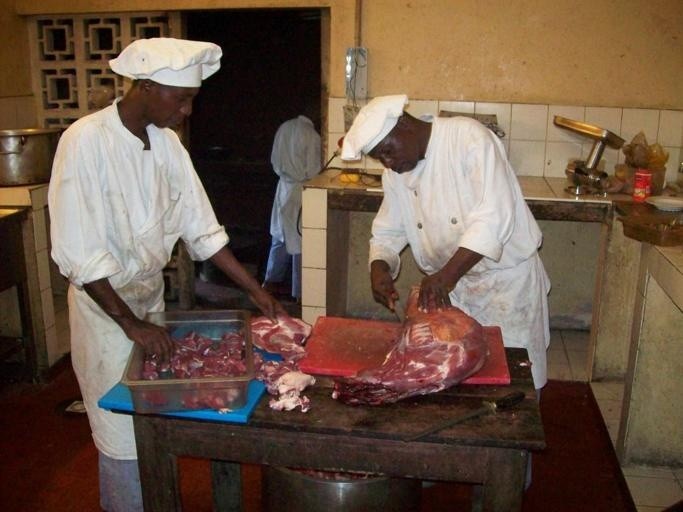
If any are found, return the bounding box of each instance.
[342,95,552,494]
[48,36,293,512]
[261,101,321,304]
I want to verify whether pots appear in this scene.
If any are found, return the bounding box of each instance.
[0,128,61,186]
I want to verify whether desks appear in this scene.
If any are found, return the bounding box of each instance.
[110,334,546,512]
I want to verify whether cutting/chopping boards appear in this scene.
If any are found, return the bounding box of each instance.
[298,315,512,386]
[97,315,288,424]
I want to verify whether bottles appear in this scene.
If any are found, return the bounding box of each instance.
[633,168,652,201]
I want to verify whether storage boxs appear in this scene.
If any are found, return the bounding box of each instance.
[121,309,252,409]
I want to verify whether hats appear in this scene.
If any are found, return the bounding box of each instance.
[108,37,223,88]
[341,94,409,161]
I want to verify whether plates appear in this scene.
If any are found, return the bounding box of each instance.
[645,195,683,212]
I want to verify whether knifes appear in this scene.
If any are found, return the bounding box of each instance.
[390,296,407,323]
